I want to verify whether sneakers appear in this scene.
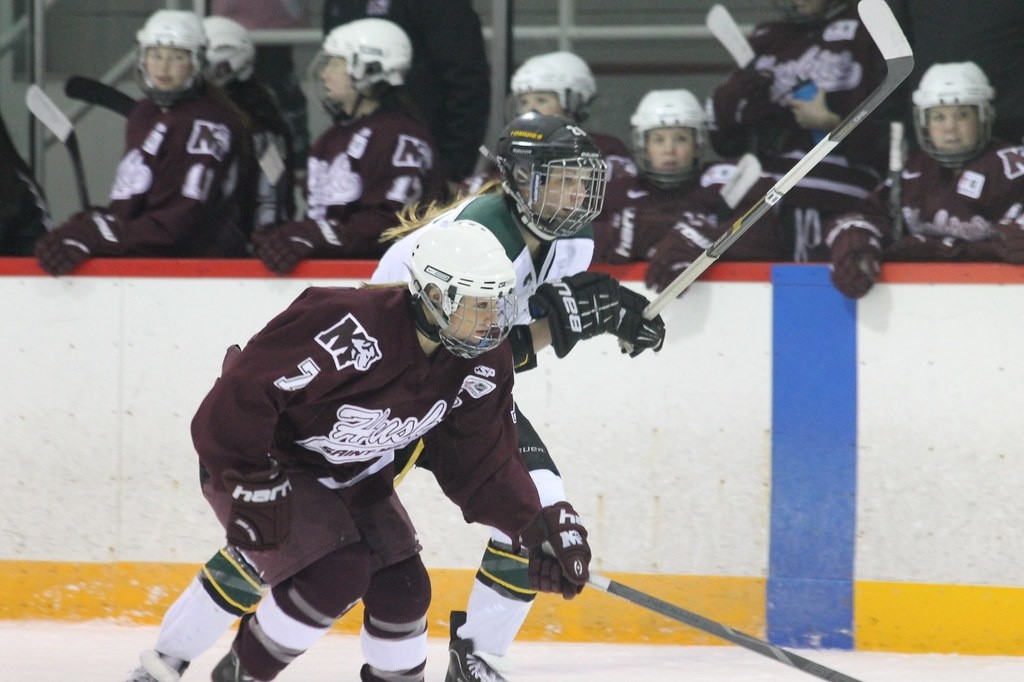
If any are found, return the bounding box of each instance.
[212,613,264,682]
[445,610,508,682]
[125,650,189,682]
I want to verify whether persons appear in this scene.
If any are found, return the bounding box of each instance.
[706,1,883,255]
[459,55,768,298]
[0,0,490,275]
[127,114,665,682]
[826,62,1024,296]
[189,220,591,682]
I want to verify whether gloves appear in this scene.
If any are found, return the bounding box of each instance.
[964,217,1024,264]
[644,220,715,296]
[251,216,343,275]
[226,454,294,551]
[610,286,666,358]
[826,220,885,301]
[528,271,622,358]
[725,68,774,129]
[37,206,126,274]
[511,501,591,600]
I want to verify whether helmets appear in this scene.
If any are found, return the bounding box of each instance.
[630,90,708,192]
[407,218,517,361]
[509,51,596,112]
[135,9,208,67]
[322,19,414,81]
[487,111,609,241]
[911,62,994,166]
[200,17,255,80]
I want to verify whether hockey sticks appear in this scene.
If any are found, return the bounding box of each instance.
[63,74,137,119]
[586,570,862,682]
[258,139,307,220]
[617,0,916,352]
[24,83,92,214]
[881,121,904,259]
[705,3,762,159]
